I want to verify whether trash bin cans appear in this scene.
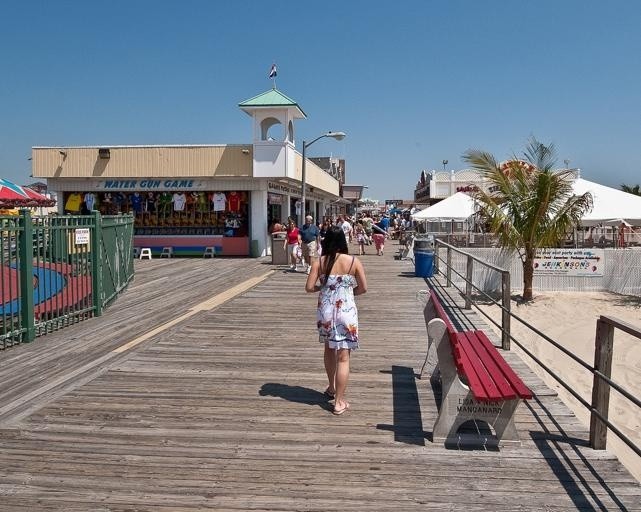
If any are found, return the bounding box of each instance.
[271,231,291,265]
[413,240,435,277]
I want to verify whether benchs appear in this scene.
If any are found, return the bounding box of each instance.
[421,290,535,447]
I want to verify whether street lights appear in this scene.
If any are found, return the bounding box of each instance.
[563,159,570,169]
[440,159,448,170]
[301,128,347,226]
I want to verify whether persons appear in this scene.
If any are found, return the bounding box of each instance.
[317,209,412,258]
[296,215,320,275]
[283,220,301,270]
[304,226,369,414]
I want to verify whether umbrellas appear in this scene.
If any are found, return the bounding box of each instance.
[1,178,56,210]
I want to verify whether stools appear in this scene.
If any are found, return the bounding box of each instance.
[133,246,217,259]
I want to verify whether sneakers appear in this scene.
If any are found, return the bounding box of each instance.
[288,258,312,275]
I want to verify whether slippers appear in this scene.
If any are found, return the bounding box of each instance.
[326,384,336,398]
[333,400,351,415]
